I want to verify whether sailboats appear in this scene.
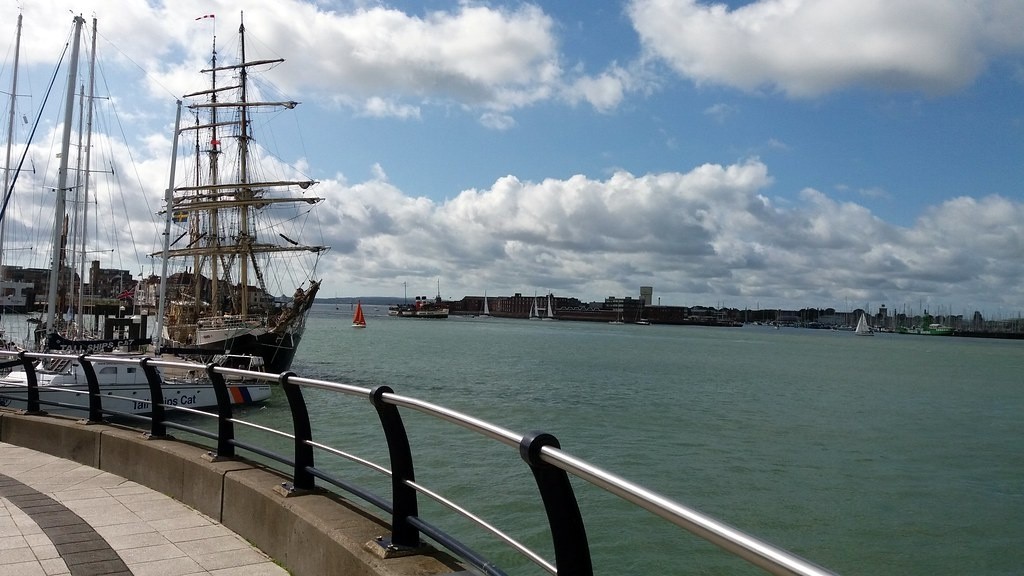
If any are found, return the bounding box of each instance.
[608,298,625,325]
[634,296,651,325]
[854,311,876,336]
[350,298,368,328]
[529,288,543,321]
[0,1,330,422]
[541,290,557,321]
[478,288,492,318]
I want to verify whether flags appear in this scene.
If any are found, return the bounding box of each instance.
[115,284,136,302]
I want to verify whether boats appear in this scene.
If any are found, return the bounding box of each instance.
[387,278,450,318]
